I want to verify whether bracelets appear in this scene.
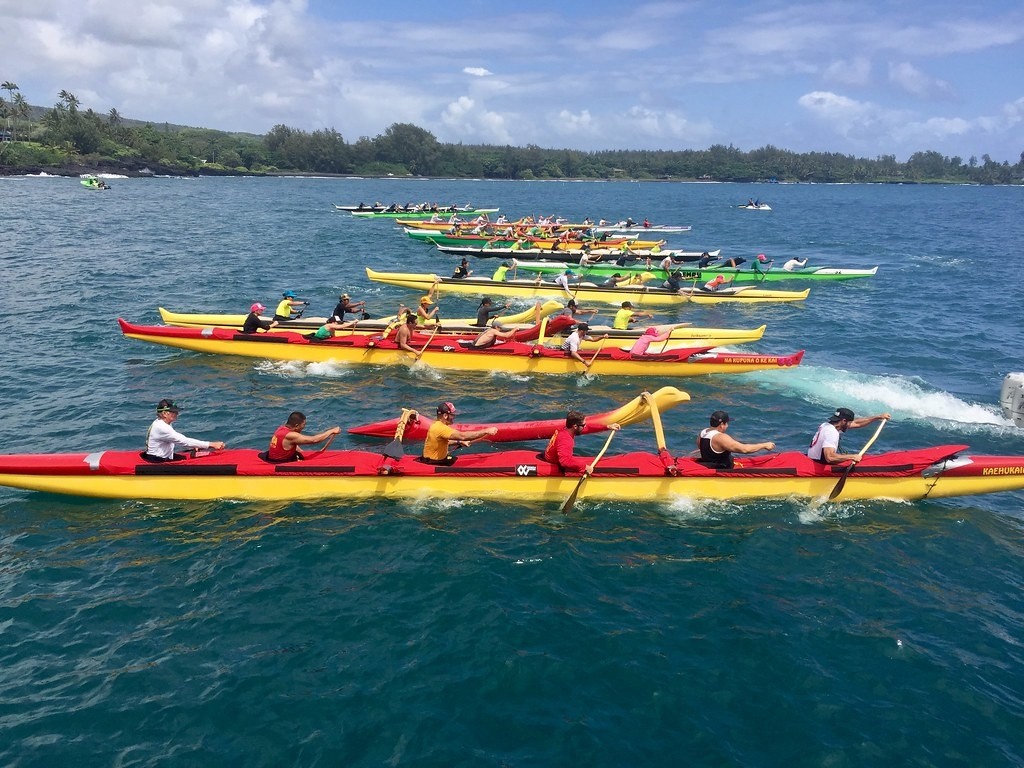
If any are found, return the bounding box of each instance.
[583,361,586,364]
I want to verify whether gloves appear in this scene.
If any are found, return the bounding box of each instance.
[305,302,310,305]
[297,310,302,315]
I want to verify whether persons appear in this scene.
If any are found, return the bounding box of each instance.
[545,411,621,477]
[267,412,342,462]
[698,410,775,468]
[142,399,225,462]
[806,407,892,465]
[422,402,498,467]
[243,199,809,368]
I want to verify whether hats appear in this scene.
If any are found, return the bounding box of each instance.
[156,398,184,412]
[251,303,267,313]
[568,299,581,309]
[710,411,735,426]
[645,328,660,336]
[828,408,854,422]
[462,237,802,279]
[491,321,503,327]
[622,301,635,309]
[436,402,462,415]
[327,315,343,323]
[578,323,592,331]
[339,294,352,301]
[419,297,434,304]
[284,291,298,298]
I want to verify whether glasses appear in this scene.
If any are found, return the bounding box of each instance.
[577,423,587,427]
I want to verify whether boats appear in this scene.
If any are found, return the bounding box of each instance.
[505,258,879,283]
[153,174,174,178]
[729,203,772,211]
[117,318,805,373]
[435,243,722,262]
[80,176,109,190]
[394,217,693,247]
[156,300,768,352]
[365,265,810,305]
[331,203,501,217]
[1,385,1024,502]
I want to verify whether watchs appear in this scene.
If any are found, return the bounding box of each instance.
[457,440,461,445]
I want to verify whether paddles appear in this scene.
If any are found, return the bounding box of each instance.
[828,417,886,501]
[586,312,596,323]
[436,281,442,333]
[561,426,619,515]
[660,331,671,354]
[450,434,490,453]
[182,447,213,453]
[415,326,438,361]
[295,305,307,318]
[691,448,700,455]
[497,307,509,316]
[364,201,808,299]
[582,338,605,374]
[629,316,654,323]
[320,433,337,452]
[352,322,357,335]
